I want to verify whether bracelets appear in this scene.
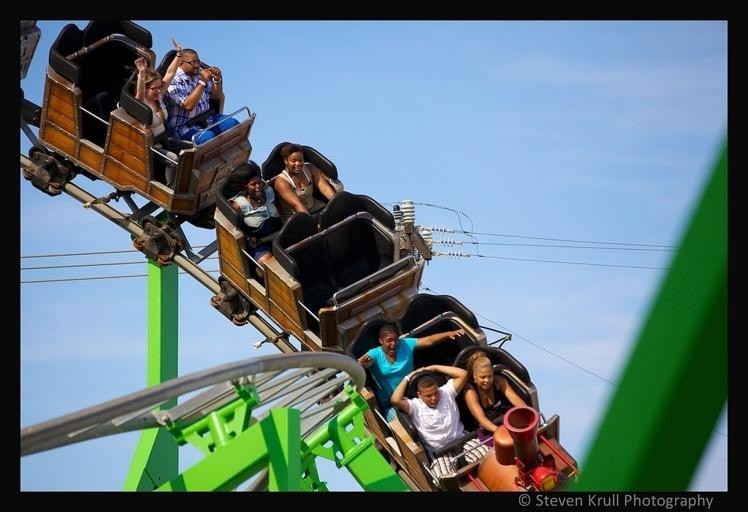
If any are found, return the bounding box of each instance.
[212,76,222,84]
[175,52,184,58]
[403,374,410,383]
[138,73,147,80]
[198,80,207,87]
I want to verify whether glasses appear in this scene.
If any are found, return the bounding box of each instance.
[184,60,195,65]
[148,86,163,91]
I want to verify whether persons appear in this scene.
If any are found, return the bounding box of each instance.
[133,36,194,190]
[162,48,240,146]
[356,323,465,424]
[227,159,284,282]
[390,363,492,489]
[460,350,528,448]
[273,144,337,226]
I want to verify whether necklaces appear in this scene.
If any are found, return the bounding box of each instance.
[246,193,266,203]
